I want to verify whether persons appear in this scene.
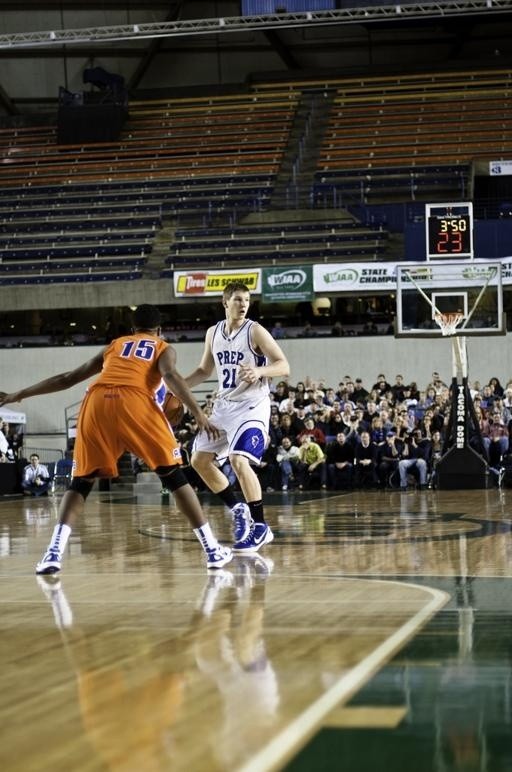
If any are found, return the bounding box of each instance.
[194,552,283,772]
[169,282,292,555]
[131,369,512,498]
[0,417,22,465]
[34,567,235,772]
[20,453,52,495]
[0,303,234,574]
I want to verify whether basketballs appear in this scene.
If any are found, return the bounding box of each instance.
[162,393,184,426]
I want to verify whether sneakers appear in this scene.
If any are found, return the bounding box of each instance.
[282,485,288,493]
[248,552,274,579]
[35,575,60,591]
[205,544,234,569]
[35,549,63,575]
[266,486,275,493]
[236,561,254,589]
[322,486,327,491]
[206,570,234,588]
[298,485,304,491]
[232,522,275,553]
[230,502,252,543]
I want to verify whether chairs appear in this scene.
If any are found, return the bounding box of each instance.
[51,459,72,494]
[325,435,337,443]
[468,437,512,489]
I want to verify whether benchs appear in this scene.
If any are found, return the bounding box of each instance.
[0,69,512,284]
